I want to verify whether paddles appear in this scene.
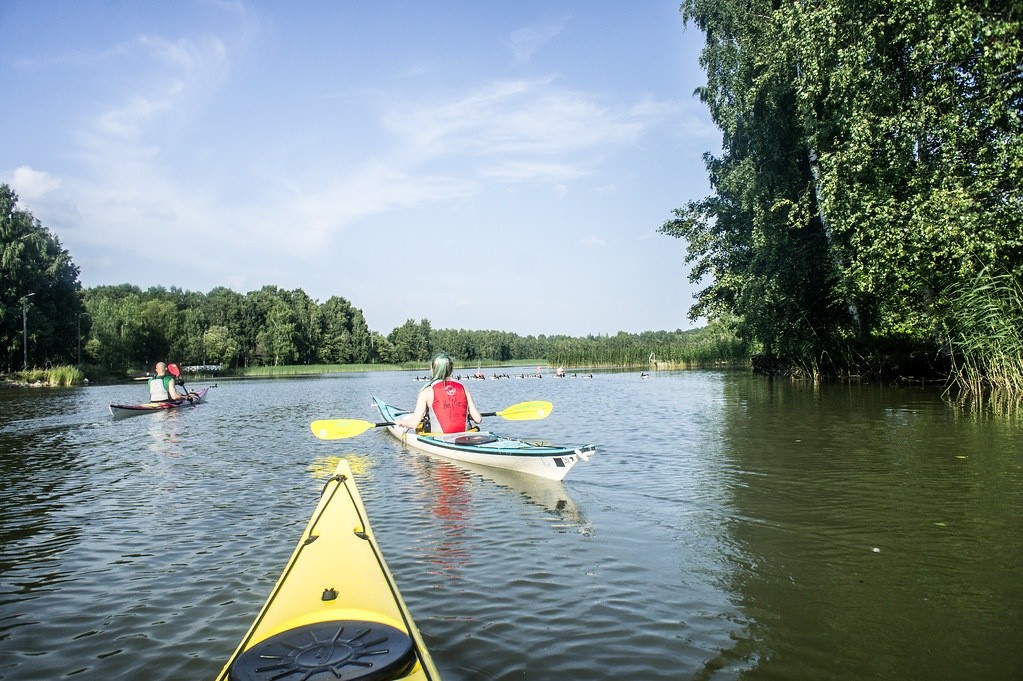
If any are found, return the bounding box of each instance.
[310,400,553,439]
[168,363,196,406]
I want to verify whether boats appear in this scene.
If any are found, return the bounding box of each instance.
[109,387,210,419]
[211,456,441,681]
[369,394,597,481]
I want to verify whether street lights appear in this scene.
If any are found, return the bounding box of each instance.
[23,293,36,368]
[77,312,87,364]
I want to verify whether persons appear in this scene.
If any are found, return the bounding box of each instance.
[147,361,195,403]
[390,352,482,438]
